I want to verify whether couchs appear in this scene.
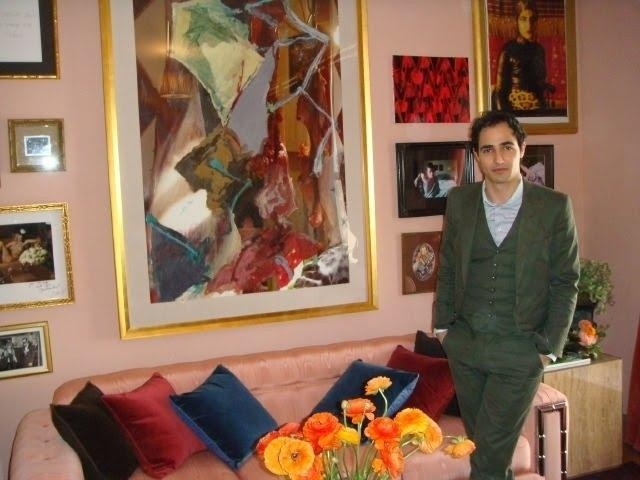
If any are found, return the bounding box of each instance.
[8,332,567,480]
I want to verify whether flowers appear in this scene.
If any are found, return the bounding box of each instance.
[253,371,475,480]
[566,255,615,364]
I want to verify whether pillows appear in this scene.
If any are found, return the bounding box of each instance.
[41,378,139,479]
[414,330,463,419]
[97,370,206,480]
[171,362,275,473]
[385,344,454,422]
[312,358,419,445]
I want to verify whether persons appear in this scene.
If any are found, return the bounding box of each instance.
[2,337,35,369]
[430,109,581,479]
[0,231,40,264]
[493,1,549,112]
[413,163,439,200]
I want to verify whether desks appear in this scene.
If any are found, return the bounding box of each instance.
[542,349,629,479]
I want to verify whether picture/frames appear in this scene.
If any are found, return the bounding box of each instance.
[470,0,579,137]
[98,0,379,344]
[520,142,555,187]
[0,0,61,79]
[0,320,53,381]
[394,140,473,217]
[7,116,66,174]
[1,199,77,313]
